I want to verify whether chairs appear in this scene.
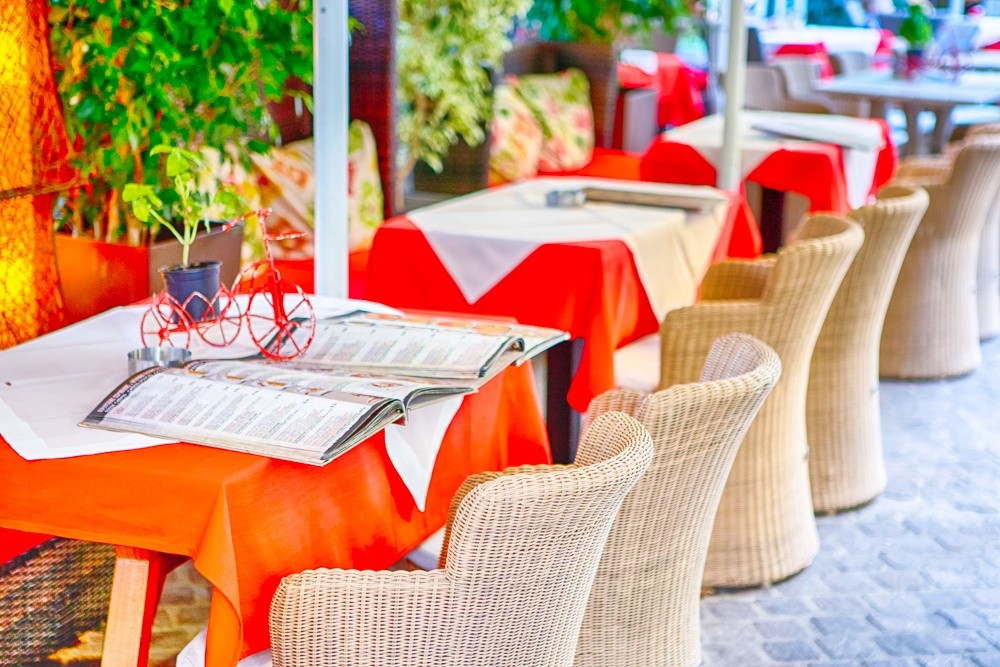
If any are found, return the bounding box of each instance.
[180,51,1000,667]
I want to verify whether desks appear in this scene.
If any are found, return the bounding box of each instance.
[811,71,1000,156]
[369,178,737,469]
[0,294,554,667]
[652,112,897,251]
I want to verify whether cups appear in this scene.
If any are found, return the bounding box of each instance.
[126,346,192,377]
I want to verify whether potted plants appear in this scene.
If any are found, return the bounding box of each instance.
[46,0,361,325]
[124,145,245,323]
[518,0,705,152]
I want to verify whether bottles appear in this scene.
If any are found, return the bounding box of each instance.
[900,7,934,51]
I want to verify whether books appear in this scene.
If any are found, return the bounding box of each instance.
[750,116,891,151]
[75,304,572,471]
[574,183,724,212]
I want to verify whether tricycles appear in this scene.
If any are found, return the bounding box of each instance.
[138,208,317,363]
